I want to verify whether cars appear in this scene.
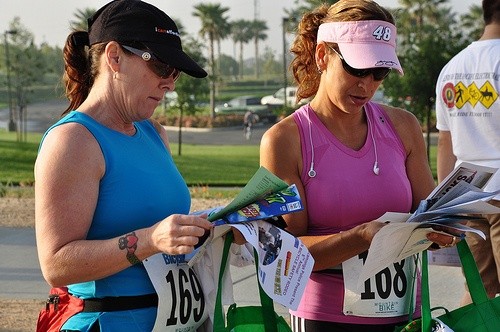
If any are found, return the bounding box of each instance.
[261,87,311,110]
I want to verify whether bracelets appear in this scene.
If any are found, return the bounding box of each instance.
[340,230,348,242]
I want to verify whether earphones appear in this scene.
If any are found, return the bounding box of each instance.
[373,161,380,175]
[308,162,317,178]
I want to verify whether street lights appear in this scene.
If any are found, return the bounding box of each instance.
[281,16,298,119]
[3,29,20,132]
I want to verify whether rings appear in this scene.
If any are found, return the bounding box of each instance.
[444,237,456,247]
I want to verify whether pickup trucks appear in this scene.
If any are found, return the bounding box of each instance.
[215,96,280,113]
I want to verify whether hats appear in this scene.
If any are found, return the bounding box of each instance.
[87,0,208,79]
[316,20,405,76]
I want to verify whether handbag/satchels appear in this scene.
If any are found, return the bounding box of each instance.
[400,227,500,331]
[36,285,87,332]
[210,230,296,332]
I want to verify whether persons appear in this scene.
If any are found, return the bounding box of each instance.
[34,0,250,332]
[259,0,468,332]
[435,0,500,297]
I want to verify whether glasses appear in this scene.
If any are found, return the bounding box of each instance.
[325,41,392,81]
[119,44,182,83]
